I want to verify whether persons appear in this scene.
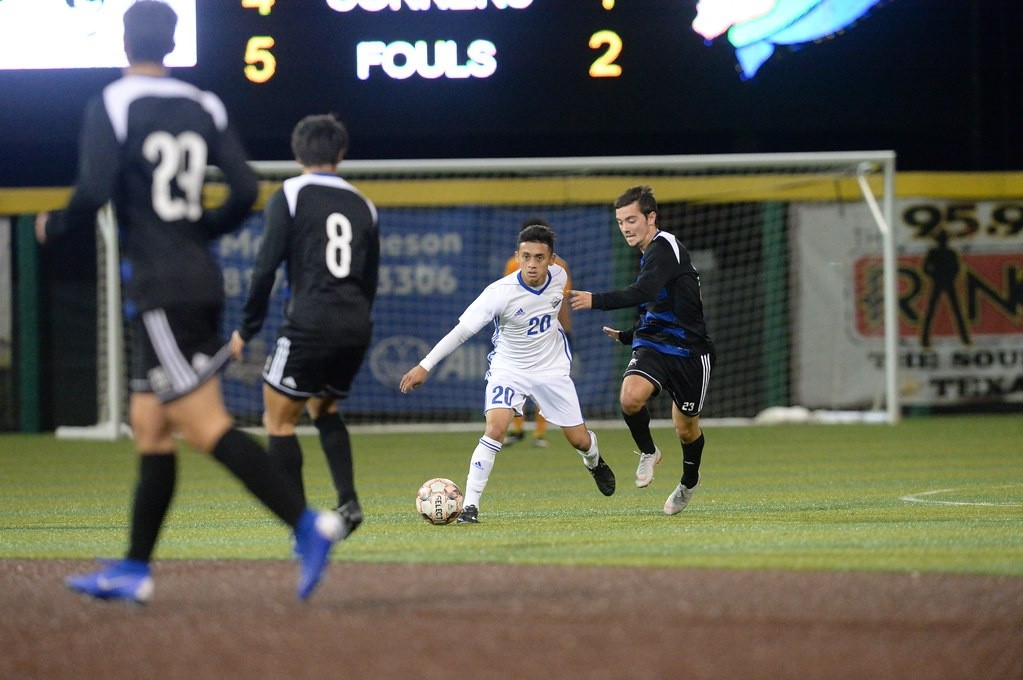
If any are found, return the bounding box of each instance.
[503,219,570,448]
[399,224,615,524]
[231,112,381,540]
[566,184,715,516]
[35,0,345,606]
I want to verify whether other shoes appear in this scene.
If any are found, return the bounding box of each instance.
[503,432,524,445]
[532,436,547,449]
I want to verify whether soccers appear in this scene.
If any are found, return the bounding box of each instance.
[416,476,465,527]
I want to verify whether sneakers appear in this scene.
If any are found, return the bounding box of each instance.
[585,454,616,496]
[457,504,478,524]
[664,470,701,516]
[335,496,362,540]
[287,505,347,598]
[634,444,662,488]
[65,560,154,606]
